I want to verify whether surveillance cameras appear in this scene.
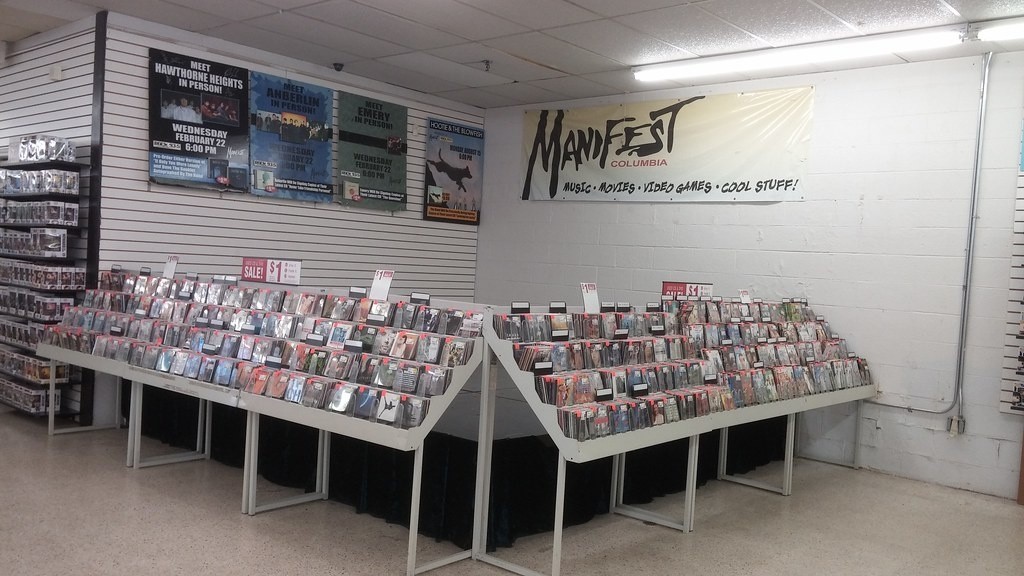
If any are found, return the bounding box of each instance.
[333,63,344,71]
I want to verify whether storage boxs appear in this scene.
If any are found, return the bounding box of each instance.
[0,169,85,412]
[7,133,77,164]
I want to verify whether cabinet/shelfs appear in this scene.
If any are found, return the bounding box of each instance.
[0,160,91,427]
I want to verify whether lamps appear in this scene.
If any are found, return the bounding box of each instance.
[969,16,1024,42]
[630,23,969,83]
